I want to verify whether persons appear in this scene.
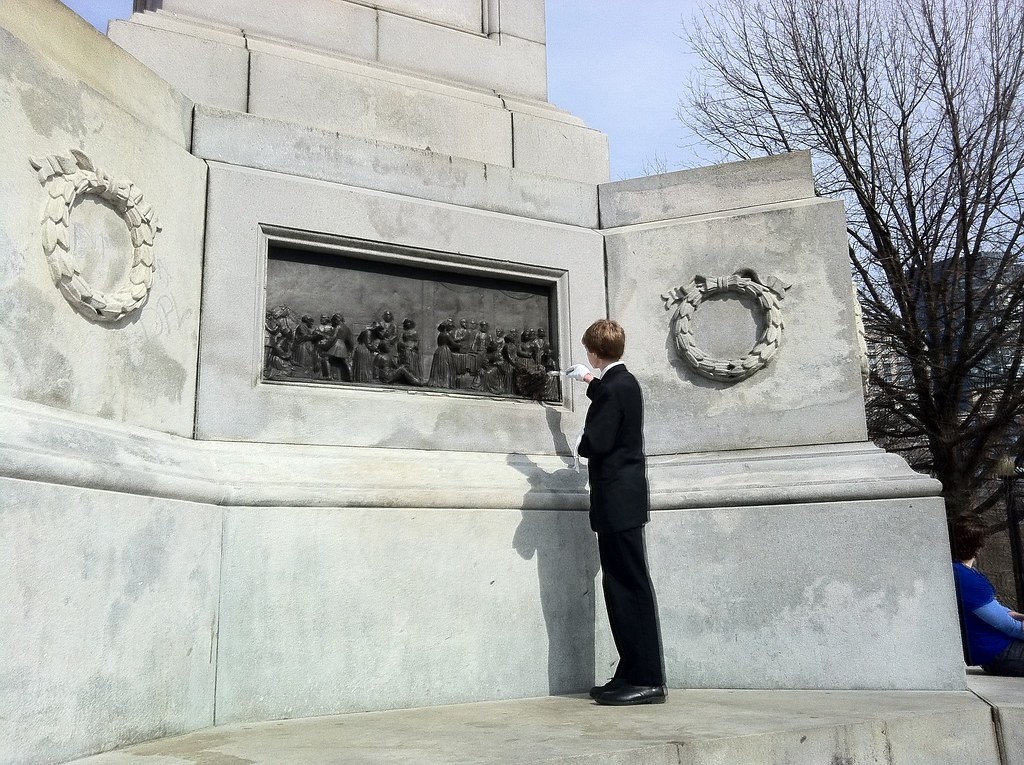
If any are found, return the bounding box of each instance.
[948,516,1024,676]
[566,319,669,706]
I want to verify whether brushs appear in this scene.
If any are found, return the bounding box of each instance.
[547,371,571,377]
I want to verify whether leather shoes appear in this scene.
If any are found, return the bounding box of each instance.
[595,683,665,704]
[590,677,668,698]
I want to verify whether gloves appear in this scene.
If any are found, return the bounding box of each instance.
[564,364,591,382]
